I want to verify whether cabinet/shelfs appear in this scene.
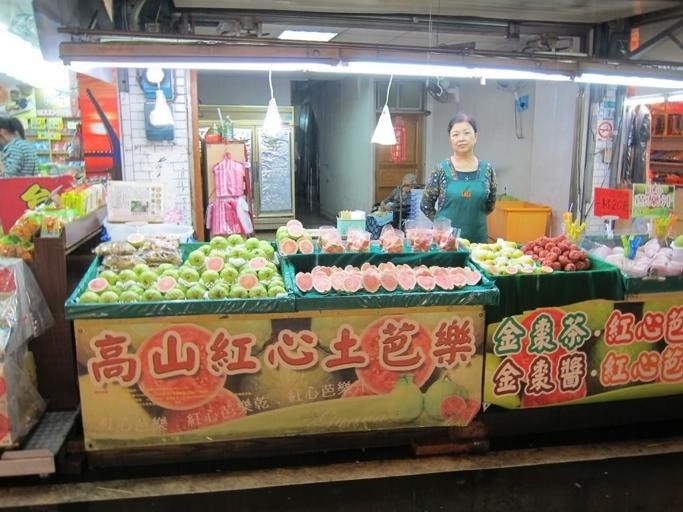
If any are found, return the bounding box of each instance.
[224,125,294,220]
[26,110,86,173]
[625,97,682,187]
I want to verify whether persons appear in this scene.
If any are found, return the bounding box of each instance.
[6,90,20,110]
[0,118,39,178]
[420,115,497,243]
[380,173,418,210]
